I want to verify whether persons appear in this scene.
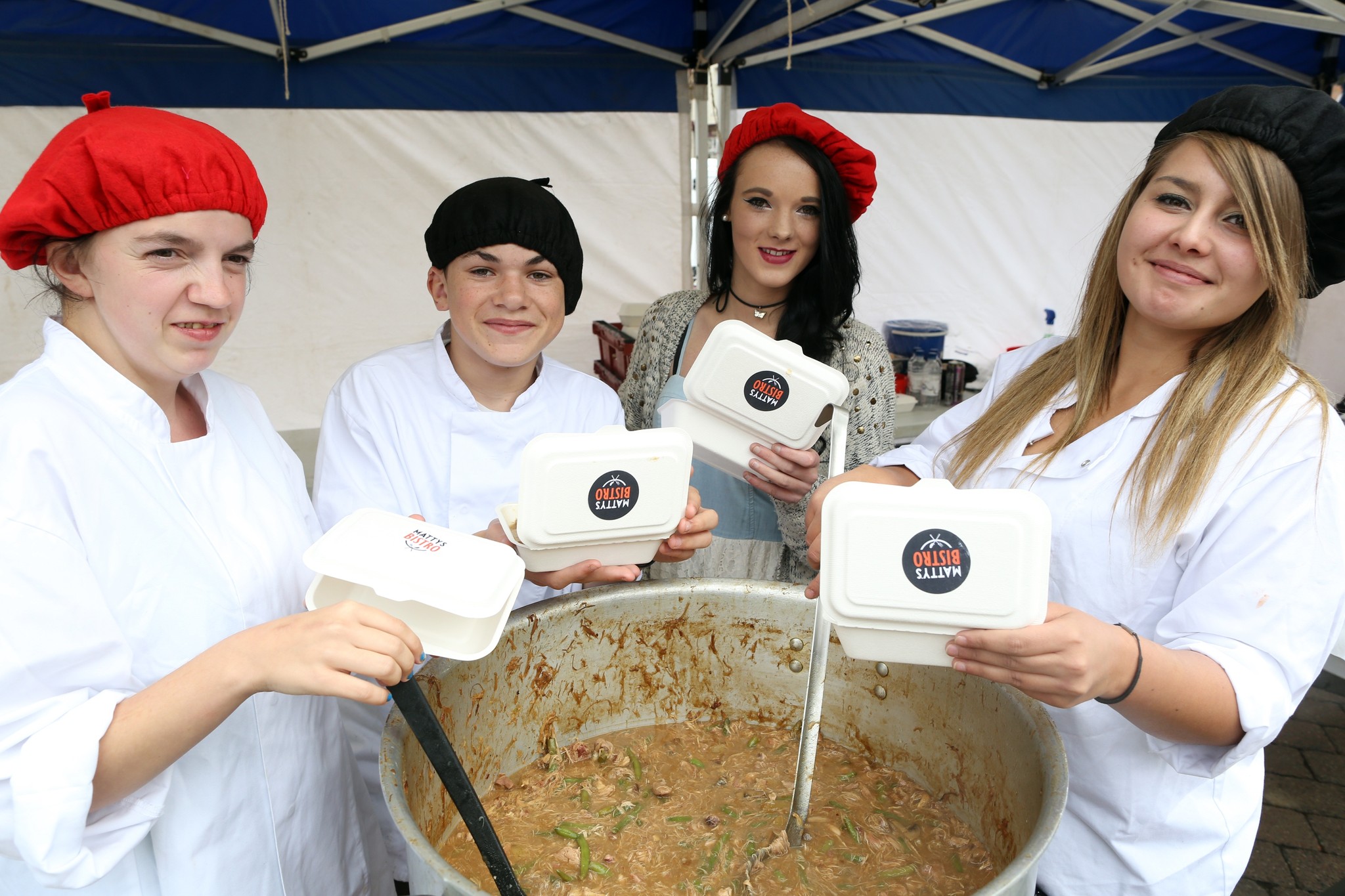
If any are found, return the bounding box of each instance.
[618,102,896,585]
[311,177,719,896]
[804,84,1345,896]
[0,92,427,896]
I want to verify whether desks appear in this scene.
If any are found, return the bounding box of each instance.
[893,391,981,444]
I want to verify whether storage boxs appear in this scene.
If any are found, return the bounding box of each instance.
[592,319,637,387]
[881,319,949,361]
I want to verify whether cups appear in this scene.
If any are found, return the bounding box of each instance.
[895,373,907,394]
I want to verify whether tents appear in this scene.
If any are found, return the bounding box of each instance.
[0,0,1345,404]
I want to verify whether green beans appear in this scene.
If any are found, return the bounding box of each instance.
[513,715,964,896]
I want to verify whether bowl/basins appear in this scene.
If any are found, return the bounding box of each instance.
[655,321,851,486]
[895,393,919,413]
[820,478,1052,668]
[493,422,693,573]
[618,301,653,339]
[304,507,526,663]
[941,359,978,396]
[377,577,1069,896]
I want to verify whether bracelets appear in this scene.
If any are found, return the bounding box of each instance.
[1094,623,1143,704]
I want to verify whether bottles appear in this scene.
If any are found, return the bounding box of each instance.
[907,346,941,408]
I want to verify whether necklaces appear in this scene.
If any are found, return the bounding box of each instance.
[729,285,786,319]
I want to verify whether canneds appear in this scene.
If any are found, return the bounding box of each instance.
[944,360,965,406]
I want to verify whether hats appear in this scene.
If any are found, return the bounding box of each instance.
[0,89,267,275]
[717,102,877,225]
[1150,85,1345,300]
[424,176,584,315]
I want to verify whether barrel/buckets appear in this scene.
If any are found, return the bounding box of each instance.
[881,319,948,359]
